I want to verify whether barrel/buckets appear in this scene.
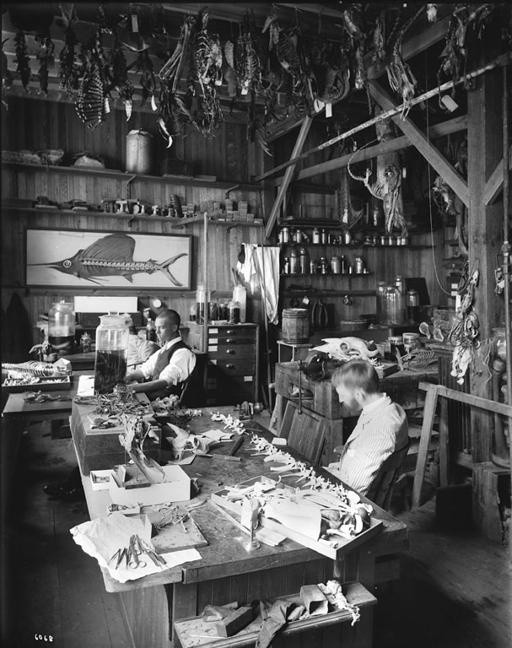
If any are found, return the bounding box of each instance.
[282,308,311,344]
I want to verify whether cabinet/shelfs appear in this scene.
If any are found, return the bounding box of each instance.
[278,220,411,296]
[0,149,266,230]
[185,326,259,407]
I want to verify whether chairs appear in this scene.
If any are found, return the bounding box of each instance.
[364,435,411,509]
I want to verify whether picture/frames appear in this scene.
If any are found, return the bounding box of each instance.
[23,228,192,291]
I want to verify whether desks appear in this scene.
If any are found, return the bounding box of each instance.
[69,405,411,648]
[0,367,153,507]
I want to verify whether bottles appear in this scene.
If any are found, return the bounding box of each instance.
[210,297,242,325]
[377,275,419,324]
[93,315,127,397]
[46,300,76,348]
[279,229,369,276]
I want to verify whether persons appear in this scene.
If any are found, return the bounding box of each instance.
[123,309,201,403]
[321,360,408,502]
